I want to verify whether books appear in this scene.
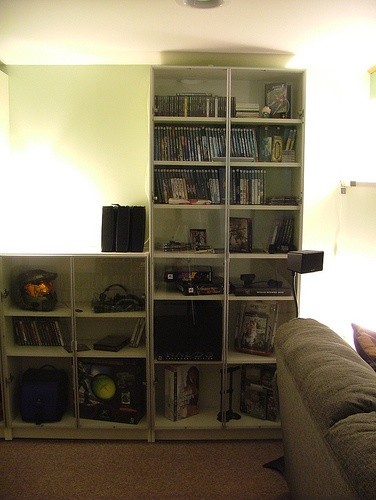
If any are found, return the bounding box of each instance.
[12,317,145,352]
[229,214,297,254]
[164,266,292,296]
[239,364,277,421]
[165,365,201,422]
[153,81,291,117]
[154,166,299,205]
[234,303,279,355]
[163,229,209,252]
[154,123,296,162]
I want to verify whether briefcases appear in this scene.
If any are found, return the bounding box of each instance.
[20,365,68,425]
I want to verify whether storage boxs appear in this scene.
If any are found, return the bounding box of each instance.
[20,368,69,425]
[164,365,201,422]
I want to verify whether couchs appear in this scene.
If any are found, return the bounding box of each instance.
[275,317,376,500]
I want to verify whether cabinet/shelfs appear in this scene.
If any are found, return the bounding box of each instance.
[0,66,307,442]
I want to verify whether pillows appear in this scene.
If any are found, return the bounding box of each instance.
[351,322,376,372]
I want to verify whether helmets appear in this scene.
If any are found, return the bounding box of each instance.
[15,270,58,312]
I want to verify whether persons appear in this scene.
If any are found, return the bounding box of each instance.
[243,317,264,348]
[193,231,205,250]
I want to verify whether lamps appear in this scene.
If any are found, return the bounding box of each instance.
[181,0,223,9]
